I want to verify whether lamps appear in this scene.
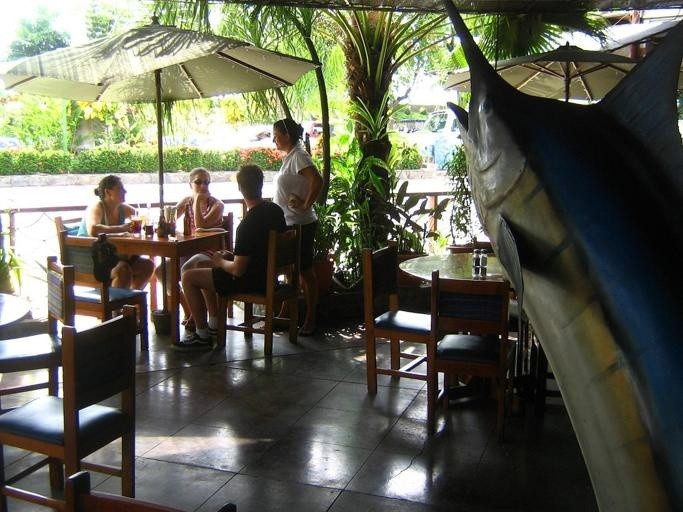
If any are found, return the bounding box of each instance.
[181,211,234,318]
[215,223,303,357]
[57,229,150,350]
[59,467,240,511]
[0,255,74,414]
[0,302,137,512]
[54,215,85,235]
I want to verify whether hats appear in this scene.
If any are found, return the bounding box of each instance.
[389,116,425,142]
[408,109,467,168]
[202,120,336,156]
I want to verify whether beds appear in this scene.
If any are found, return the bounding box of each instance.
[444,41,683,103]
[0,11,323,313]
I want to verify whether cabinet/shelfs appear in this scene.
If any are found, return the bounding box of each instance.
[472,248,480,268]
[480,266,486,280]
[480,248,487,267]
[471,265,479,279]
[157,202,194,238]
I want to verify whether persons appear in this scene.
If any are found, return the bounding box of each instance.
[259,117,323,337]
[170,164,286,352]
[77,175,156,326]
[155,167,227,330]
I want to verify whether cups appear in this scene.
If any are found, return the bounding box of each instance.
[194,180,210,185]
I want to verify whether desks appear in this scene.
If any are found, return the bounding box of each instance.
[399,253,515,291]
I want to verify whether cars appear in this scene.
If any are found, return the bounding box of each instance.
[130,208,153,239]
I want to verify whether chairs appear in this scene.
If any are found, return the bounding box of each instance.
[0,302,137,512]
[171,333,214,351]
[0,255,74,414]
[427,269,516,441]
[57,229,150,350]
[188,327,218,339]
[59,467,240,511]
[181,211,234,318]
[215,223,303,357]
[54,215,85,235]
[298,323,315,336]
[529,331,566,445]
[362,240,431,395]
